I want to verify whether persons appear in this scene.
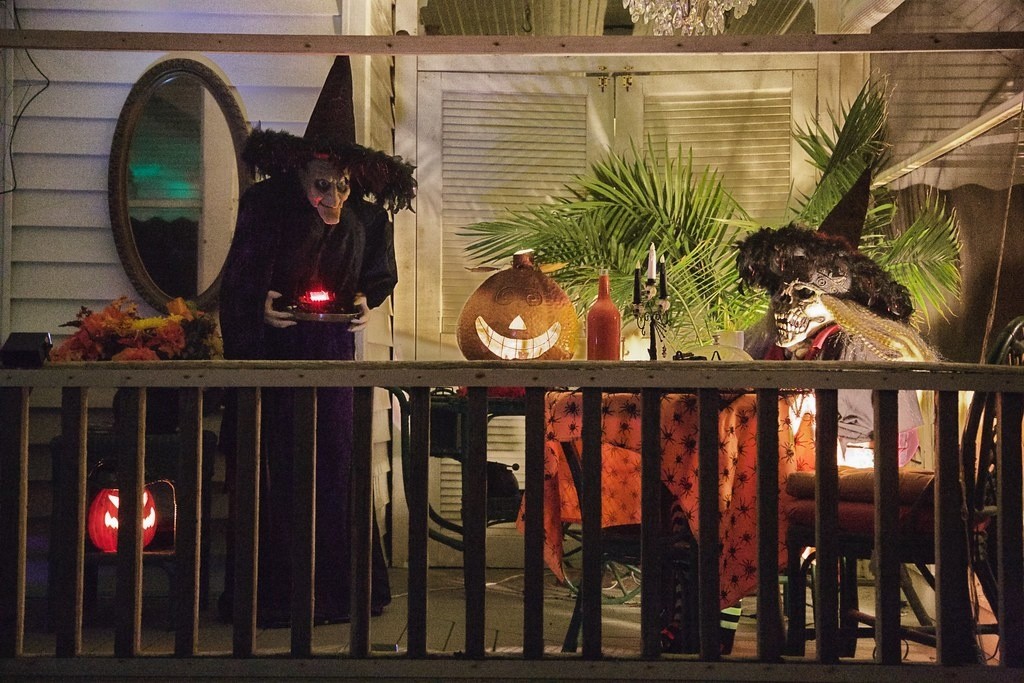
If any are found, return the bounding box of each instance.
[660,170,924,658]
[218,55,417,625]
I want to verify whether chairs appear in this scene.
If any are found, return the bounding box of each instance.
[46,428,217,654]
[784,316,1024,658]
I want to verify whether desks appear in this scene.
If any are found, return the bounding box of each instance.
[514,390,806,654]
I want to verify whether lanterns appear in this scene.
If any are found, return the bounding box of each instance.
[89,488,156,552]
[457,249,578,361]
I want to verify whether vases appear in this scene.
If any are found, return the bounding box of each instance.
[112,385,178,434]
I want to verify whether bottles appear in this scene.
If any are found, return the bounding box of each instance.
[587,269,621,360]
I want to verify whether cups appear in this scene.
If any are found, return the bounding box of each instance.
[719,331,744,350]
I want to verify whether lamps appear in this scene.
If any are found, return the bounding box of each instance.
[622,0,756,36]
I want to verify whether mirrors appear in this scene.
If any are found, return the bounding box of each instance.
[106,59,254,314]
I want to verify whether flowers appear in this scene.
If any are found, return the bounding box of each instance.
[48,296,224,362]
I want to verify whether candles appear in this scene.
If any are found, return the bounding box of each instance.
[633,260,641,302]
[647,241,656,279]
[660,254,667,299]
